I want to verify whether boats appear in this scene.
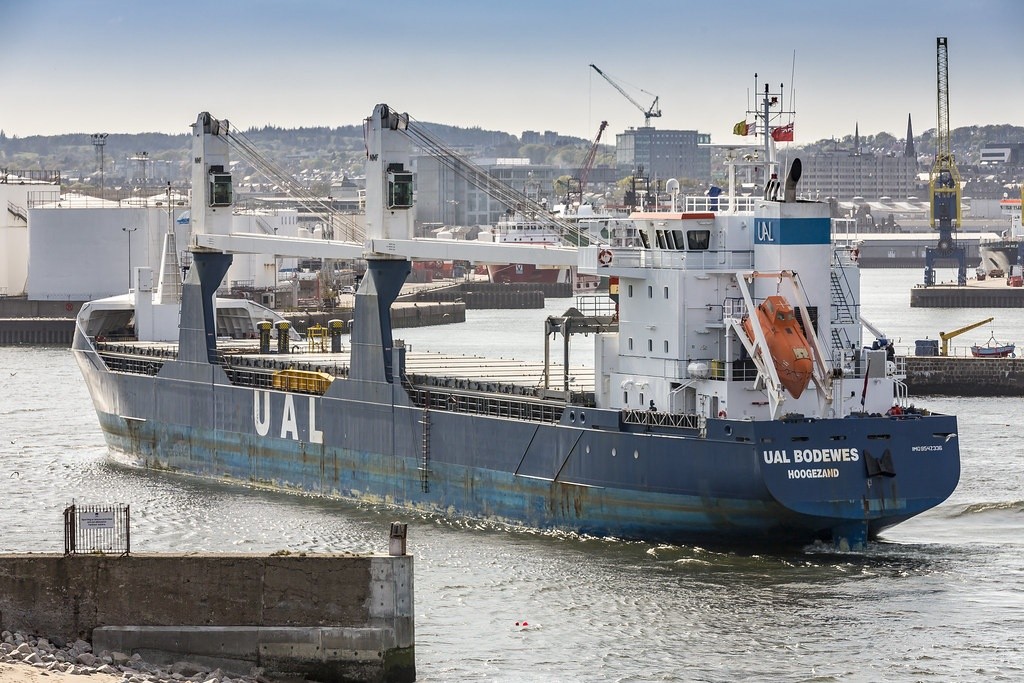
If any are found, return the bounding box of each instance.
[72,49,960,555]
[970,345,1015,358]
[743,296,813,399]
[486,262,570,284]
[493,218,560,243]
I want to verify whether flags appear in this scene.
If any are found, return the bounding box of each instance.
[771,126,782,138]
[746,122,756,135]
[771,121,794,141]
[733,120,746,136]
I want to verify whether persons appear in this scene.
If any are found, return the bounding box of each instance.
[885,405,897,415]
[894,402,902,415]
[905,402,916,414]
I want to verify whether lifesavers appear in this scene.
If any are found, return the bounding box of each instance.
[599,251,612,264]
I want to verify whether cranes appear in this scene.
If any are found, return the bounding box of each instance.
[923,36,968,287]
[590,64,662,127]
[940,318,994,356]
